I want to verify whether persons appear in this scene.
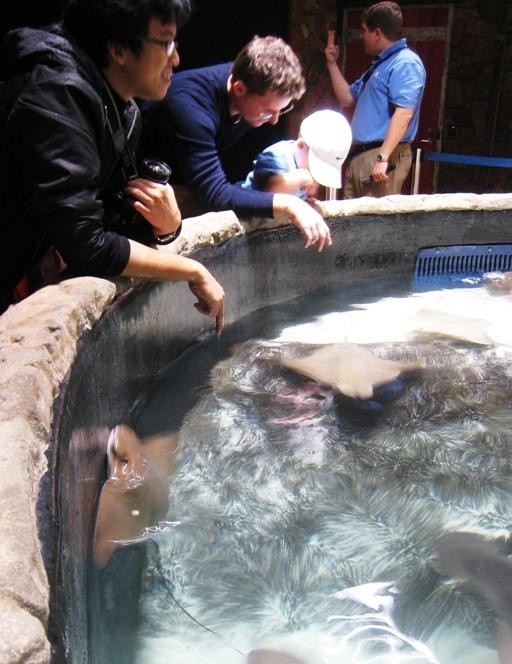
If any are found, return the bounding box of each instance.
[324,1,427,199]
[234,108,352,201]
[135,33,334,253]
[0,0,226,337]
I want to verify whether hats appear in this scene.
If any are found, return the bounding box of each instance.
[299,109,353,189]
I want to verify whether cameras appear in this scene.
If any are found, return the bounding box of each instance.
[104,157,173,245]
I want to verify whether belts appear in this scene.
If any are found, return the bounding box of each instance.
[352,141,384,155]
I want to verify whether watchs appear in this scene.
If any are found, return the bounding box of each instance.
[375,153,388,163]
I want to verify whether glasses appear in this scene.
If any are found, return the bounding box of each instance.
[257,102,294,123]
[140,37,178,57]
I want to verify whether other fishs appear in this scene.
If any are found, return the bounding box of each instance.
[92,425,224,641]
[281,342,421,402]
[426,530,512,664]
